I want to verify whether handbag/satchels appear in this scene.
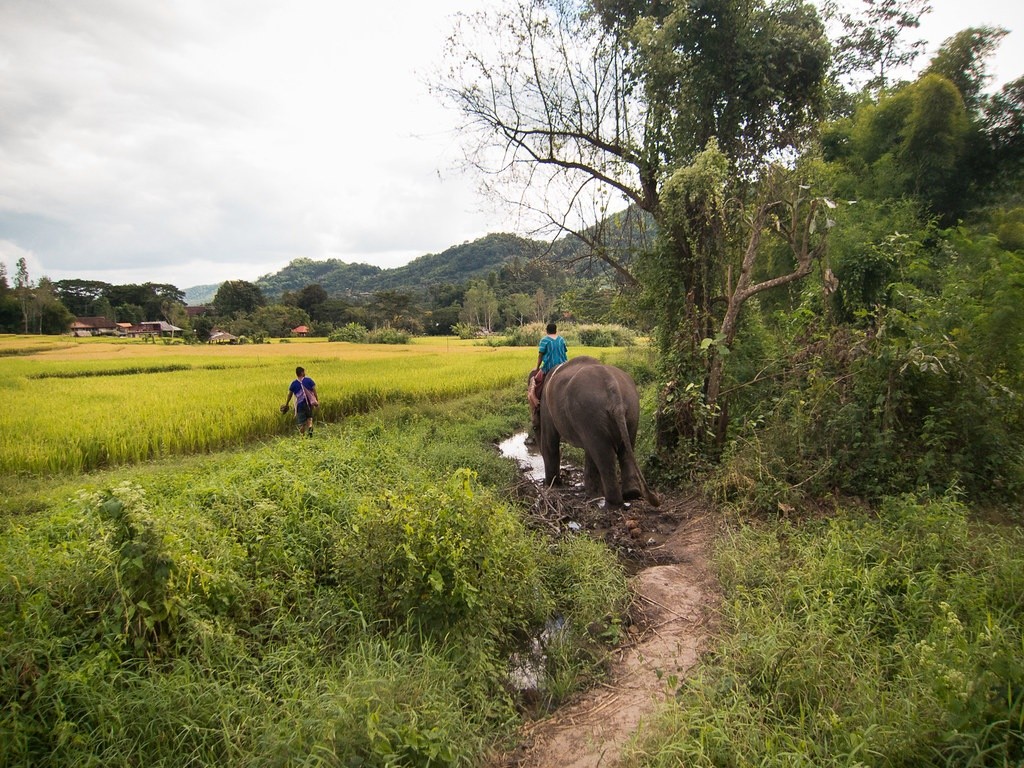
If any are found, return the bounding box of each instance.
[294,377,319,408]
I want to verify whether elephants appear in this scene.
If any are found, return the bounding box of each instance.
[527,355,661,514]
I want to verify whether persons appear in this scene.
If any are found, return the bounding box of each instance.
[533,324,568,385]
[285,366,318,438]
